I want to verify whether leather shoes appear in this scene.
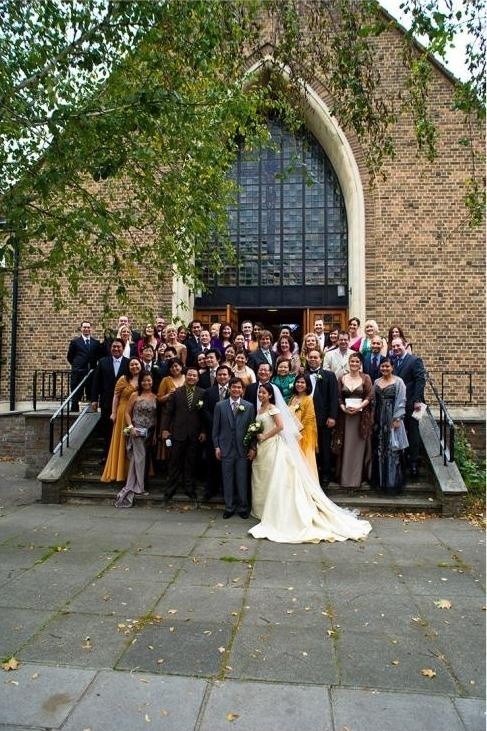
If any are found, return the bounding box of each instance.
[224,510,234,519]
[239,510,248,519]
[164,485,215,502]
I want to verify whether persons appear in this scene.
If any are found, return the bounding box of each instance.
[67,317,426,545]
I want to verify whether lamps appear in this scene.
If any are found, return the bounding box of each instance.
[189,287,202,298]
[337,285,352,297]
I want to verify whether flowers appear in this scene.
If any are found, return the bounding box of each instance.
[289,382,294,390]
[120,425,130,436]
[196,400,203,409]
[314,374,322,383]
[238,404,248,414]
[242,419,264,447]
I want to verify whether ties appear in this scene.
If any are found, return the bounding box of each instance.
[219,387,225,398]
[146,364,149,372]
[209,369,215,384]
[371,355,378,368]
[232,402,239,414]
[396,358,402,369]
[187,388,192,411]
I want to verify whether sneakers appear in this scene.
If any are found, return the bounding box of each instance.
[410,461,420,479]
[322,473,333,485]
